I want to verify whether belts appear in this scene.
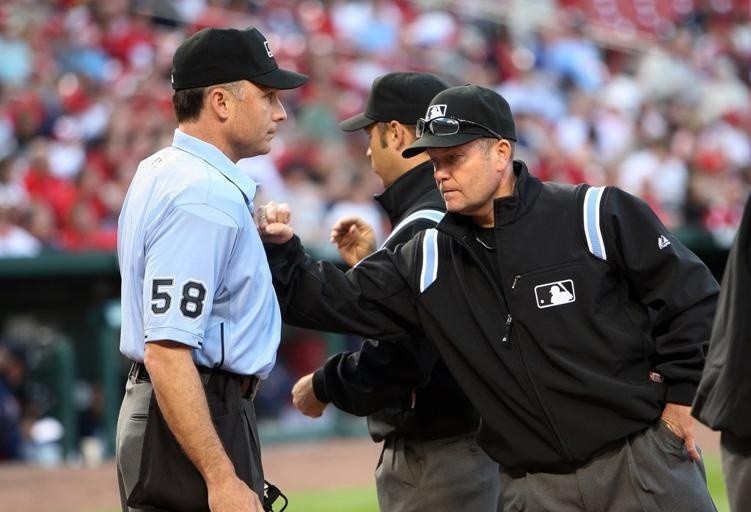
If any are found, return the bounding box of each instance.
[138,361,260,399]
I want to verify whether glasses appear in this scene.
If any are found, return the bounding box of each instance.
[416,115,502,140]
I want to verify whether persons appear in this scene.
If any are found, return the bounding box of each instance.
[329,71,504,512]
[260,84,721,512]
[690,190,751,512]
[111,26,309,512]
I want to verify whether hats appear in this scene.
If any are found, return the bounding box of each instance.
[171,26,309,90]
[339,72,452,131]
[400,83,516,159]
